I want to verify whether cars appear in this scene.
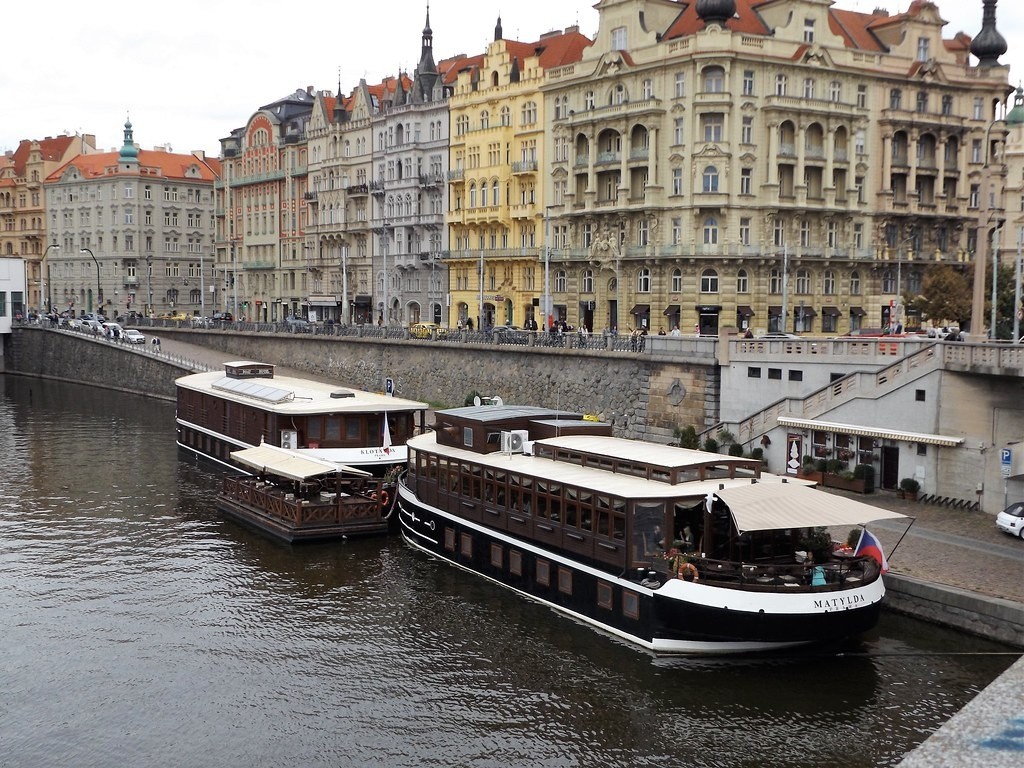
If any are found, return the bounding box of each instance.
[81,320,102,334]
[995,501,1024,540]
[28,311,210,331]
[123,329,146,344]
[212,312,233,323]
[101,322,124,338]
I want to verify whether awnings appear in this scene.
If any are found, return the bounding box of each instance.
[630,303,866,318]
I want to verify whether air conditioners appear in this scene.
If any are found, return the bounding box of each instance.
[281,431,297,442]
[281,442,297,450]
[501,430,528,453]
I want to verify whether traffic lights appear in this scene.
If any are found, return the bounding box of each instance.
[243,302,246,309]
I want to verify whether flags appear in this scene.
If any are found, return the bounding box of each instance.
[854,529,890,576]
[802,551,814,585]
[383,412,393,455]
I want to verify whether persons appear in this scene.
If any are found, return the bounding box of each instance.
[16,309,161,354]
[458,315,479,334]
[883,320,947,339]
[484,485,693,558]
[506,318,754,354]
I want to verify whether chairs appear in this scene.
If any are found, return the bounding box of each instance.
[685,535,704,556]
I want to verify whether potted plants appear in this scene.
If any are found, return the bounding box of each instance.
[667,424,768,473]
[797,455,875,494]
[900,478,920,500]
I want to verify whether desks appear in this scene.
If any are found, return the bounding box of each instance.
[787,565,832,584]
[832,549,864,578]
[707,563,732,572]
[742,568,774,585]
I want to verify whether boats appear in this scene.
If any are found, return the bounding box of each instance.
[396,393,887,658]
[174,360,430,487]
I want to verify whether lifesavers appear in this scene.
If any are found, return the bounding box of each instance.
[381,491,389,505]
[370,494,378,509]
[678,563,699,583]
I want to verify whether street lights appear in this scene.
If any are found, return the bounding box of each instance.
[39,243,60,314]
[79,248,104,314]
